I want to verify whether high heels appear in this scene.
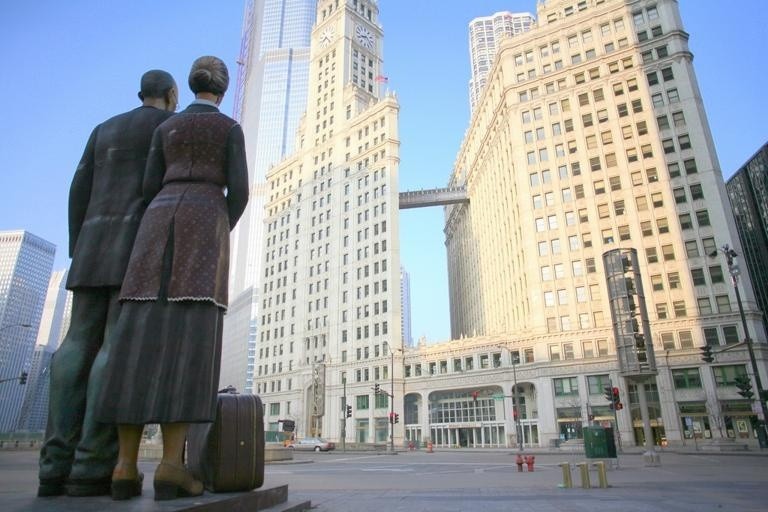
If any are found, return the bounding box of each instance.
[152,462,204,503]
[110,469,146,502]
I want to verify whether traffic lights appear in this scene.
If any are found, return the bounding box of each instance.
[700,343,715,363]
[347,405,352,418]
[732,376,755,398]
[388,412,400,424]
[373,383,382,396]
[604,387,623,411]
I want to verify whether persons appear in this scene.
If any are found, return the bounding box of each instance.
[33,68,180,500]
[89,54,251,503]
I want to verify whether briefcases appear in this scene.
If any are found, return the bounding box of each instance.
[183,385,269,497]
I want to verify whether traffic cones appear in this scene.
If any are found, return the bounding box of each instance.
[425,440,435,453]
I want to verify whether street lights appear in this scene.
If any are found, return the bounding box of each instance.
[382,341,402,451]
[496,343,524,450]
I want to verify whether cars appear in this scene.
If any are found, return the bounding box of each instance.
[287,438,335,453]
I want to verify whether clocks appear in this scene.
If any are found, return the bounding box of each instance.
[319,25,337,50]
[354,24,376,51]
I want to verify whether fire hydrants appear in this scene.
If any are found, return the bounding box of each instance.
[516,454,535,473]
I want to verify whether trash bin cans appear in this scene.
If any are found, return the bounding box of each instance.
[583,426,617,458]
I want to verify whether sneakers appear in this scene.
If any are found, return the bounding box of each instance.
[69,471,144,497]
[36,477,69,497]
